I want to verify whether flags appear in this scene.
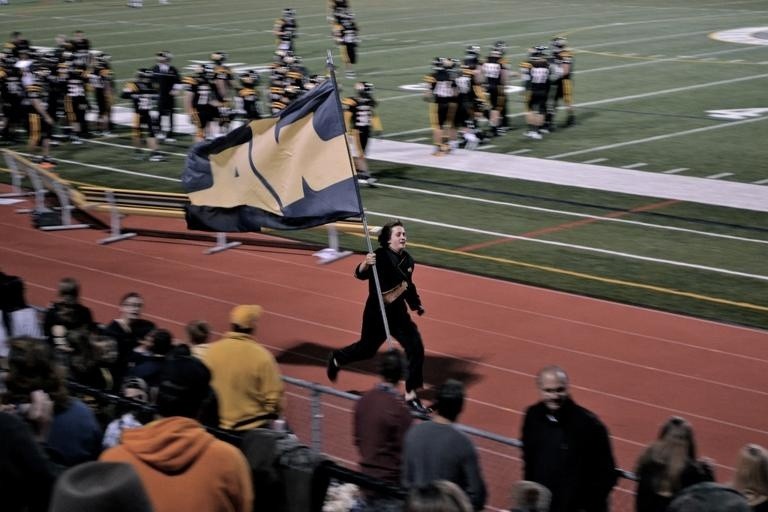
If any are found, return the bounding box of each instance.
[182,78,361,230]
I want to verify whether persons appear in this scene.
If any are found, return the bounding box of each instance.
[632,418,768,511]
[273,8,298,63]
[119,51,330,164]
[422,33,576,156]
[339,82,382,183]
[324,220,431,414]
[328,1,359,77]
[519,364,617,511]
[1,273,489,511]
[2,28,116,183]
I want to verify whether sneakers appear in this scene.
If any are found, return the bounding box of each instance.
[327,351,340,381]
[406,398,433,413]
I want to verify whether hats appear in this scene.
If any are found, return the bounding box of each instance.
[229,304,263,329]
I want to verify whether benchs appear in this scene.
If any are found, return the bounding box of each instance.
[77,184,242,255]
[310,206,385,265]
[1,148,92,230]
[0,344,451,512]
